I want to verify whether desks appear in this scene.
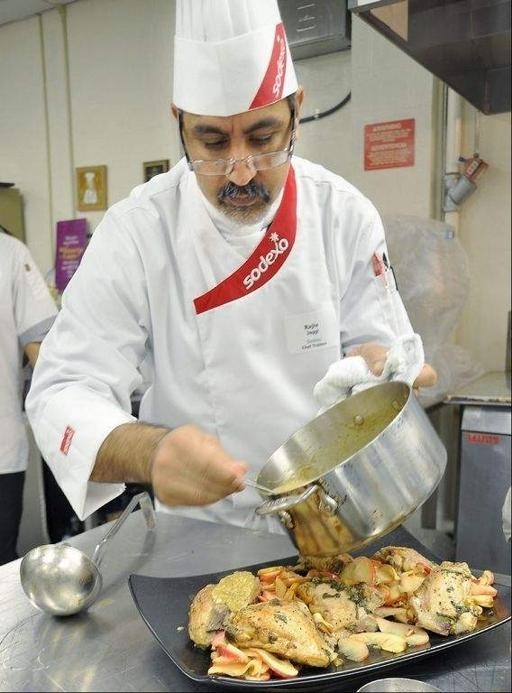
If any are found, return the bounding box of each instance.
[0,508,512,688]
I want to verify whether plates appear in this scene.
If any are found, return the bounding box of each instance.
[127,556,511,685]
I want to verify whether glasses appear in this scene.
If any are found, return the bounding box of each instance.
[174,102,295,175]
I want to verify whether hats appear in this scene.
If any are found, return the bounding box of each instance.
[171,0,299,118]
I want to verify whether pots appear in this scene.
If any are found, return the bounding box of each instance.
[254,381,447,557]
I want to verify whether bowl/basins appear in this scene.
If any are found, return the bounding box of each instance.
[357,679,442,693]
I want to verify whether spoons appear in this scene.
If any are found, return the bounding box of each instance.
[20,492,155,616]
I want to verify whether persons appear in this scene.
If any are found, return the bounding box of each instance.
[24,1,443,538]
[0,231,61,564]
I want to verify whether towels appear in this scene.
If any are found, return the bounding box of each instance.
[312,329,426,414]
